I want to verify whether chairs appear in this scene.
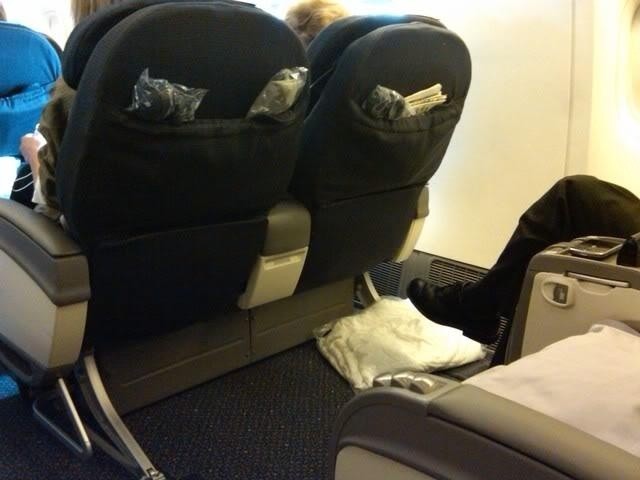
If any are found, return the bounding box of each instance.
[328,232,639,479]
[0,0,473,479]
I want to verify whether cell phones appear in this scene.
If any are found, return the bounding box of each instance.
[571,239,623,259]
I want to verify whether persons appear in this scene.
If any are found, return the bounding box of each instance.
[284,0,348,49]
[11,0,119,227]
[406,173,640,345]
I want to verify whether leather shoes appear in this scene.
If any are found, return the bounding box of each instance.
[408,278,501,345]
